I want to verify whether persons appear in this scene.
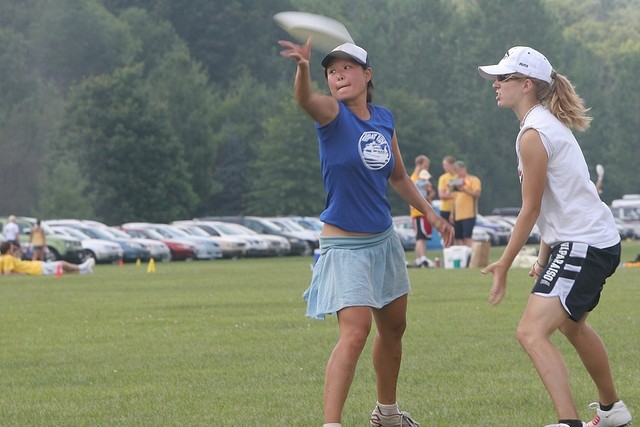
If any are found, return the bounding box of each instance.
[415,168,434,204]
[276,35,455,426]
[446,159,482,271]
[474,44,633,426]
[0,240,99,275]
[1,214,20,255]
[30,217,46,260]
[437,154,458,220]
[409,153,437,267]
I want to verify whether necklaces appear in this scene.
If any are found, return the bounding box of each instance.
[536,259,546,268]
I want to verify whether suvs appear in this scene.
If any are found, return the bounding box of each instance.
[268,218,320,256]
[494,207,541,243]
[46,220,150,260]
[200,216,309,257]
[0,217,85,262]
[488,217,514,244]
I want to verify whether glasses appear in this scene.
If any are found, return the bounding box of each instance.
[495,72,526,83]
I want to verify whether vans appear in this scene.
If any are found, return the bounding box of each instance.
[611,199,640,240]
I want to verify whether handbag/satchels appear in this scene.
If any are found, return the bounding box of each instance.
[443,245,472,269]
[467,240,491,268]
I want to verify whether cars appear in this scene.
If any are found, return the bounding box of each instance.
[175,222,250,258]
[52,227,123,264]
[215,222,270,257]
[393,216,416,250]
[477,213,500,246]
[109,226,170,260]
[119,226,198,261]
[129,223,223,260]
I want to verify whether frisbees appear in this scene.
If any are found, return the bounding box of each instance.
[273,10,356,54]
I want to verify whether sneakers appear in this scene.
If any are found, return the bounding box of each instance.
[585,401,632,427]
[84,257,95,268]
[415,259,422,268]
[88,263,95,274]
[420,256,434,268]
[369,406,431,427]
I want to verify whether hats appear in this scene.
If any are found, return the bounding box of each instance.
[419,169,431,180]
[322,42,371,69]
[477,45,555,84]
